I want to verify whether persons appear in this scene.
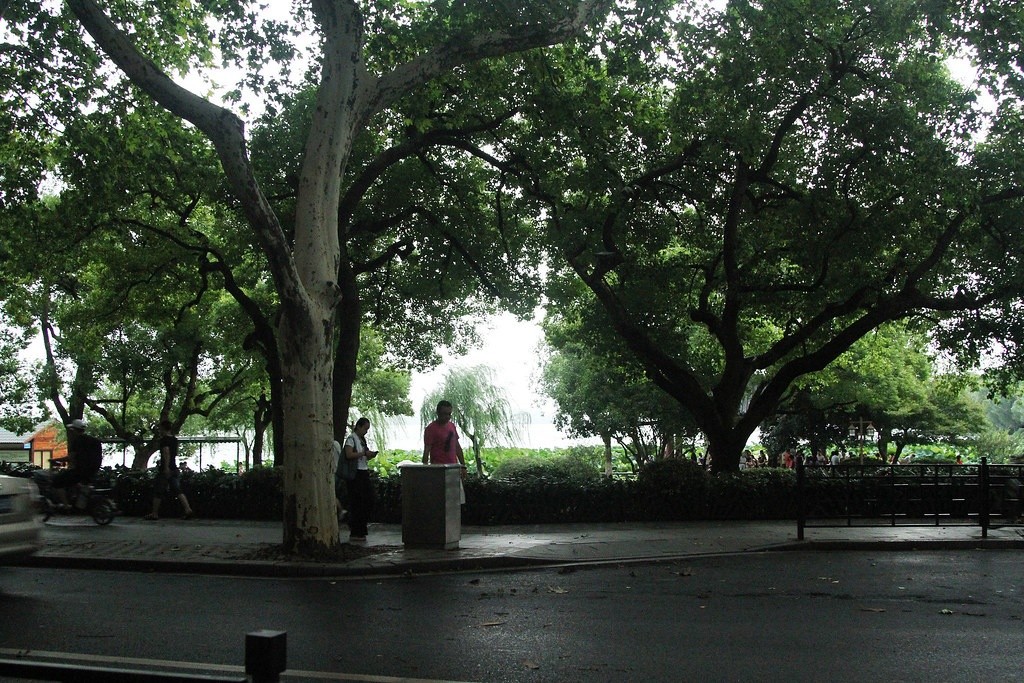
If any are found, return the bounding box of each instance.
[51,419,103,512]
[696,447,964,472]
[424,400,467,525]
[339,417,378,544]
[334,438,340,479]
[143,420,199,520]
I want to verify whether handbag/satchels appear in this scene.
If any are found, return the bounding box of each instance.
[335,437,358,481]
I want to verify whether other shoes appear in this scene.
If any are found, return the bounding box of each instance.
[350,534,366,541]
[182,511,193,520]
[339,510,348,521]
[145,514,158,520]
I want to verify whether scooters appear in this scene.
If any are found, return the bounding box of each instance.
[31,457,119,527]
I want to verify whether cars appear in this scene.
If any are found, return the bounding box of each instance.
[0,475,42,559]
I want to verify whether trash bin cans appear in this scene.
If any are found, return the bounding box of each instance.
[396,460,463,551]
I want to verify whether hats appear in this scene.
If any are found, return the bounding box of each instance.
[66,419,87,430]
